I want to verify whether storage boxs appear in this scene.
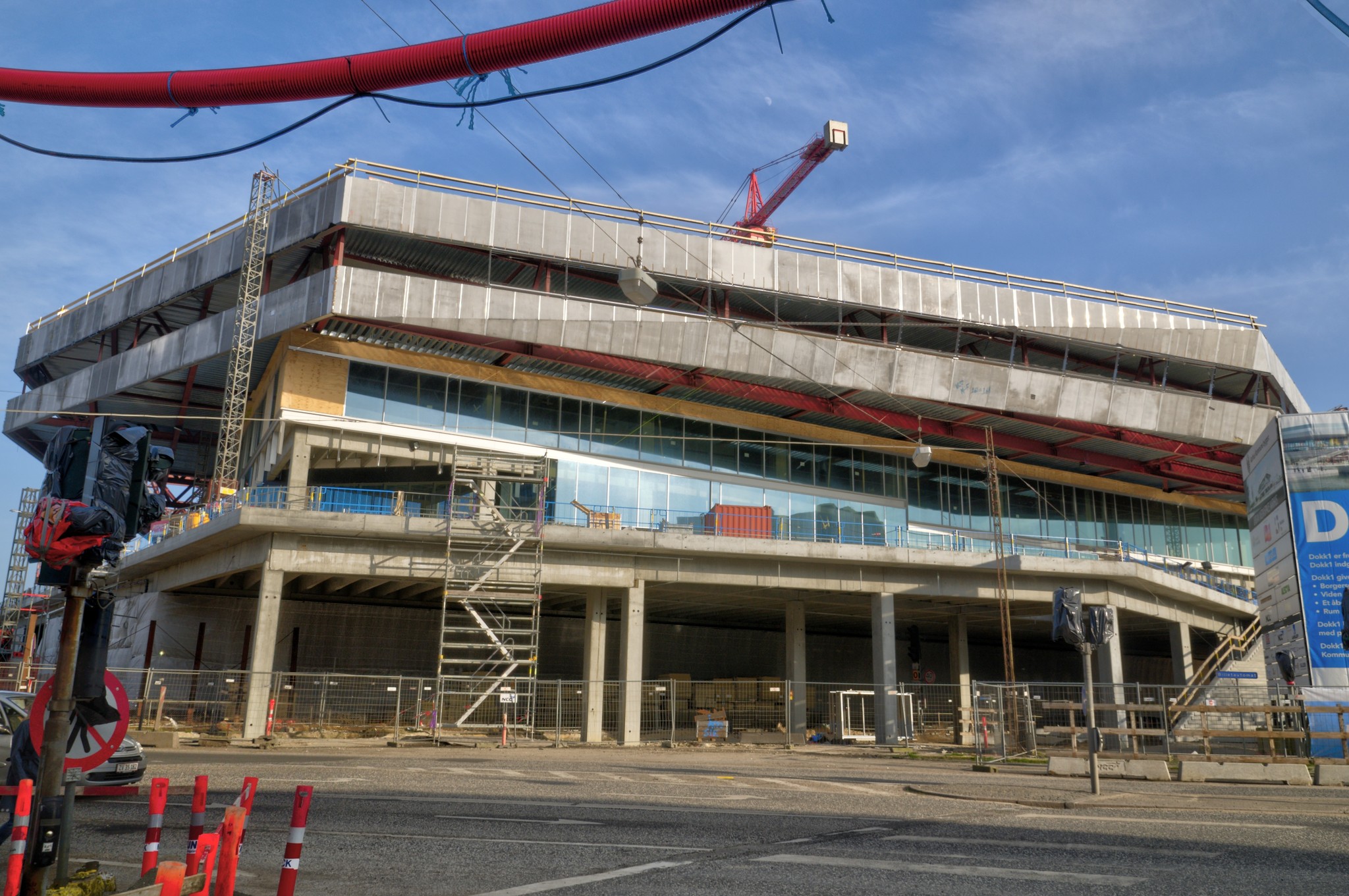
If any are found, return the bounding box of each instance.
[338,672,816,730]
[703,502,772,540]
[586,511,622,531]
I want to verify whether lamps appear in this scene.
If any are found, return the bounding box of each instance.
[616,216,659,308]
[911,414,933,469]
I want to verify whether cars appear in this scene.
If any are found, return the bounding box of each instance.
[0,690,147,788]
